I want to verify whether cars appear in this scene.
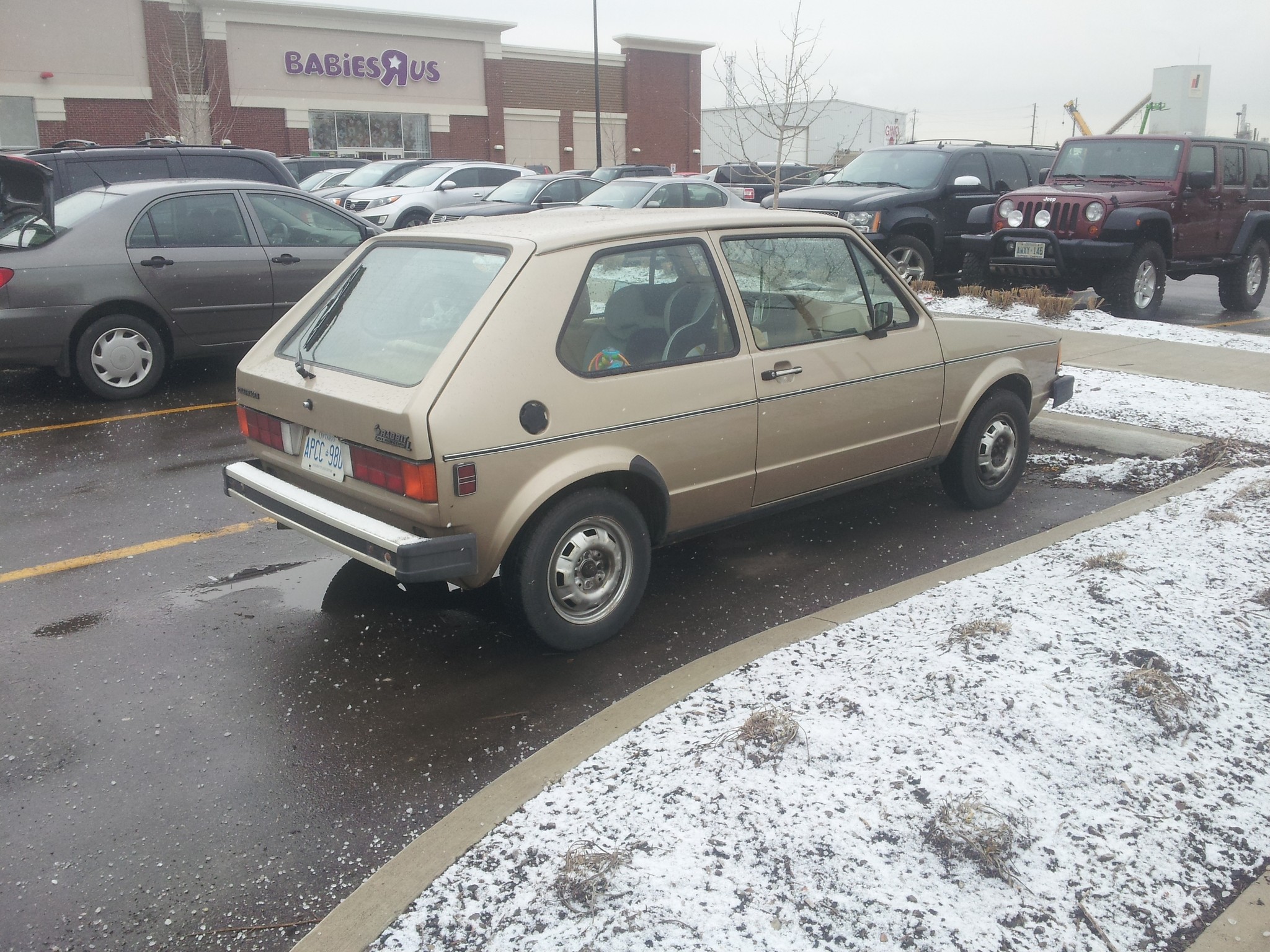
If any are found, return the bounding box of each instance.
[0,153,392,402]
[218,207,1079,654]
[279,151,848,229]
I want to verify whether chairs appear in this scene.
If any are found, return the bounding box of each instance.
[559,281,605,373]
[665,192,682,208]
[583,282,726,372]
[679,190,696,208]
[183,207,213,244]
[213,206,243,243]
[703,192,720,208]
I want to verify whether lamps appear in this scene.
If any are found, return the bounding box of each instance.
[220,139,232,145]
[693,149,701,154]
[563,146,573,152]
[494,145,504,150]
[632,148,641,152]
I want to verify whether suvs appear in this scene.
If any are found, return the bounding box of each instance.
[761,138,1060,291]
[959,133,1270,320]
[1,136,315,231]
